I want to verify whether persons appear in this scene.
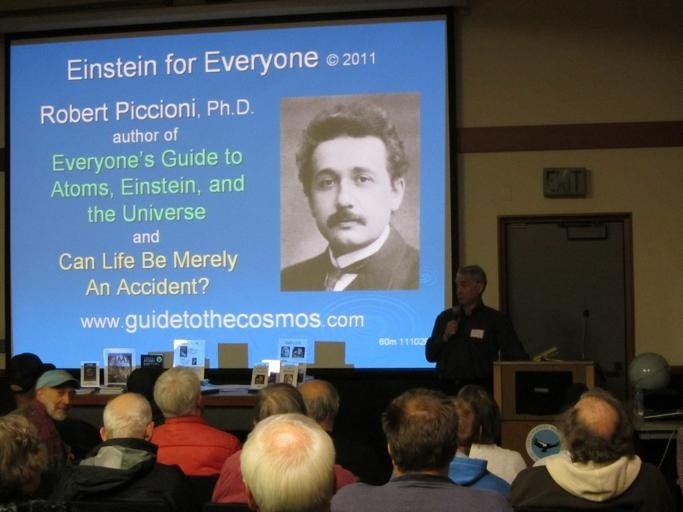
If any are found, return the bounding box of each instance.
[425,265,530,396]
[279,101,419,291]
[0,353,676,512]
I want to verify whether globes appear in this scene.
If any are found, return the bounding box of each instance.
[628,352,672,391]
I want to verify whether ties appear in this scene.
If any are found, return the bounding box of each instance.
[325,262,359,291]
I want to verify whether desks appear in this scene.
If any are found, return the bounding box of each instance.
[66,384,258,444]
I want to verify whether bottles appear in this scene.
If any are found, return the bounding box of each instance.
[631,383,644,417]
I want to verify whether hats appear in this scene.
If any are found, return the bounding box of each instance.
[7,353,55,394]
[37,368,81,393]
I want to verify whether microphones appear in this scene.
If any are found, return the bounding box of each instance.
[449,303,461,337]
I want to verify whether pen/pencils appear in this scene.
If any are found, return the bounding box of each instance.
[222,390,233,392]
